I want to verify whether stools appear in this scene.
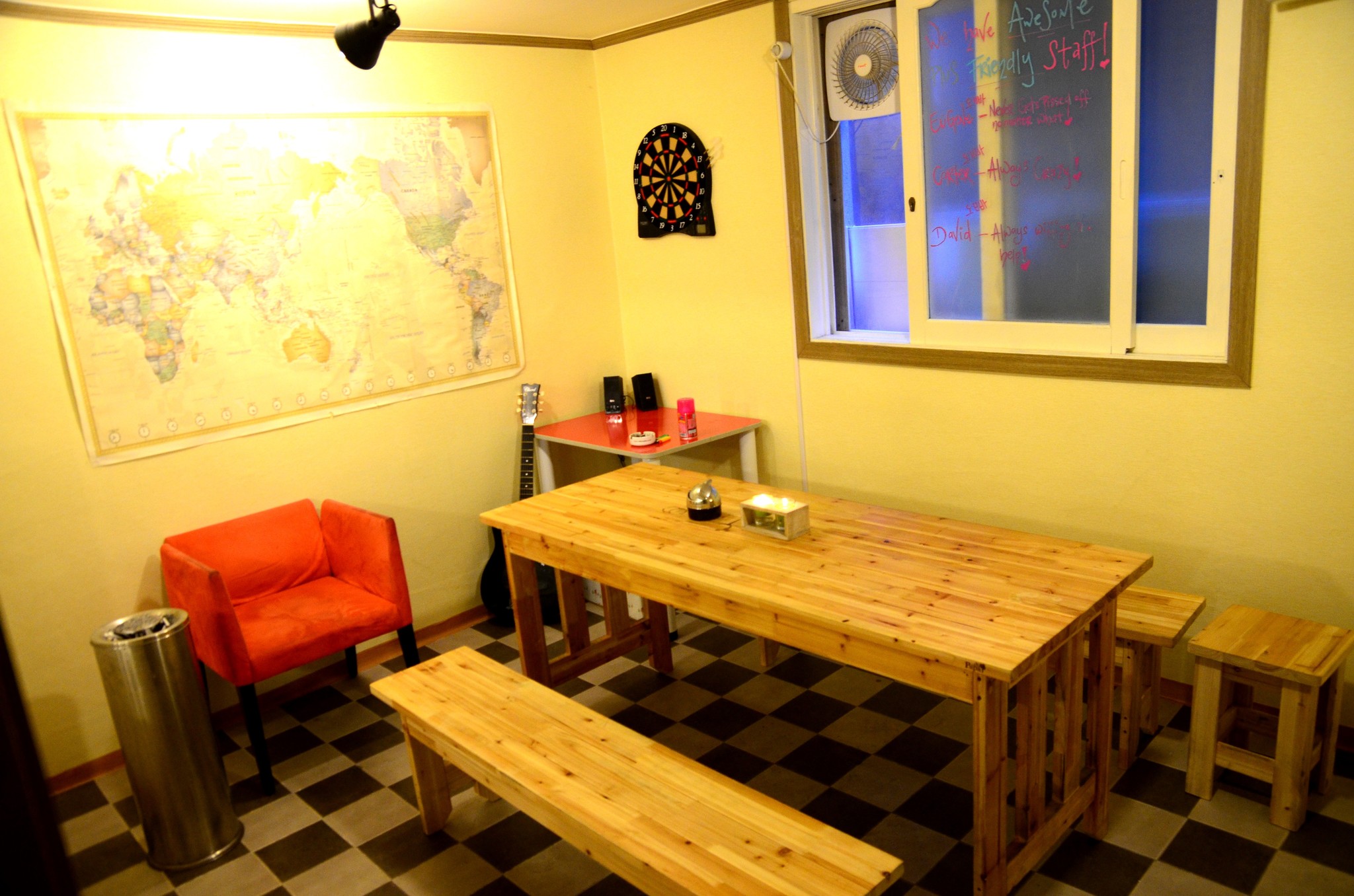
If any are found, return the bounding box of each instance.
[1183,602,1354,832]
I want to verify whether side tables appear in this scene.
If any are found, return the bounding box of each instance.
[532,404,763,639]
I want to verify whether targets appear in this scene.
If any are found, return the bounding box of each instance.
[633,122,716,237]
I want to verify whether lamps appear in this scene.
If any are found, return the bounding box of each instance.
[333,0,401,71]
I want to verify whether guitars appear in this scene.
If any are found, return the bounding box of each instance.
[478,382,565,625]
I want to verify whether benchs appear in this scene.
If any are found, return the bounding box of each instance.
[1086,582,1209,770]
[368,644,907,895]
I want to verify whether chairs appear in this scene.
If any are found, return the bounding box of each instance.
[158,496,422,796]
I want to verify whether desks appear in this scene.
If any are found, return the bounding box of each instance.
[478,461,1156,896]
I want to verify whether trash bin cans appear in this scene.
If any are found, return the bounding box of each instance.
[89,608,245,871]
